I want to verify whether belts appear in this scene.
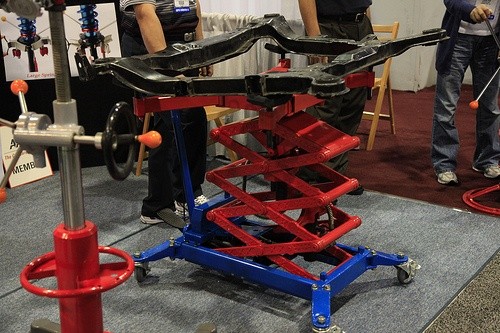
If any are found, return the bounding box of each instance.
[326,11,366,23]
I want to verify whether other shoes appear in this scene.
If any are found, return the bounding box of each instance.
[175,195,210,215]
[349,186,364,195]
[139,213,164,223]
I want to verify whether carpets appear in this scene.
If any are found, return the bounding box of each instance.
[345,82,500,218]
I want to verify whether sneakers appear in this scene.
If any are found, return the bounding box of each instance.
[472,162,500,178]
[434,169,458,184]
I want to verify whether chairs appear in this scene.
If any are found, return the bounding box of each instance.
[363,22,400,150]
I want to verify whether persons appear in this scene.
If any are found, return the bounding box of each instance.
[120,0,213,224]
[298,0,373,195]
[431,0,500,184]
